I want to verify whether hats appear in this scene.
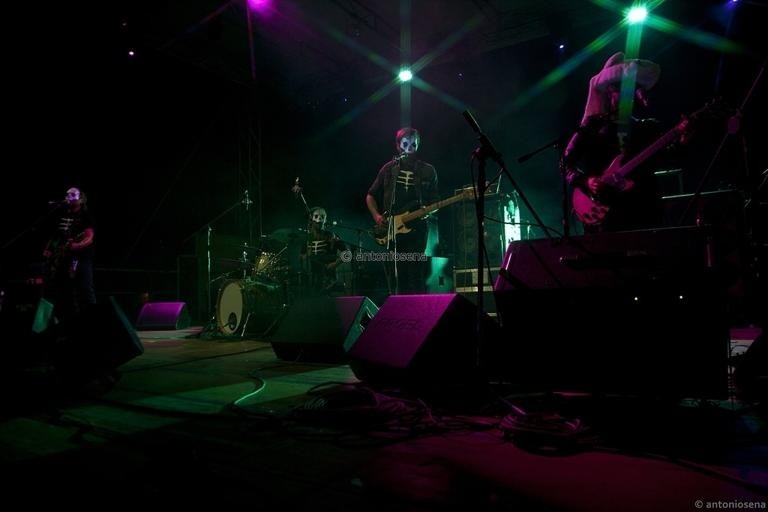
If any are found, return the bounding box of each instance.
[595,51,662,92]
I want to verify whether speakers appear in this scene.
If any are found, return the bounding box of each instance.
[136,301,192,331]
[347,292,502,409]
[267,295,380,364]
[66,295,144,370]
[494,226,728,401]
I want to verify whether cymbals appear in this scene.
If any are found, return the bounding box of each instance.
[232,244,262,252]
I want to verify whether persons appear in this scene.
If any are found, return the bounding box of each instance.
[365,127,440,297]
[42,183,99,310]
[558,50,693,233]
[299,205,349,283]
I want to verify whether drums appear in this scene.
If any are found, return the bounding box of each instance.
[216,278,286,340]
[254,252,278,279]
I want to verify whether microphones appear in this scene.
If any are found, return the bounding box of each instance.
[48,200,68,208]
[394,152,408,161]
[244,190,249,211]
[294,176,301,200]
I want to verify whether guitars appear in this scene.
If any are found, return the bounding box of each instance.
[374,181,492,245]
[572,98,720,226]
[45,229,85,280]
